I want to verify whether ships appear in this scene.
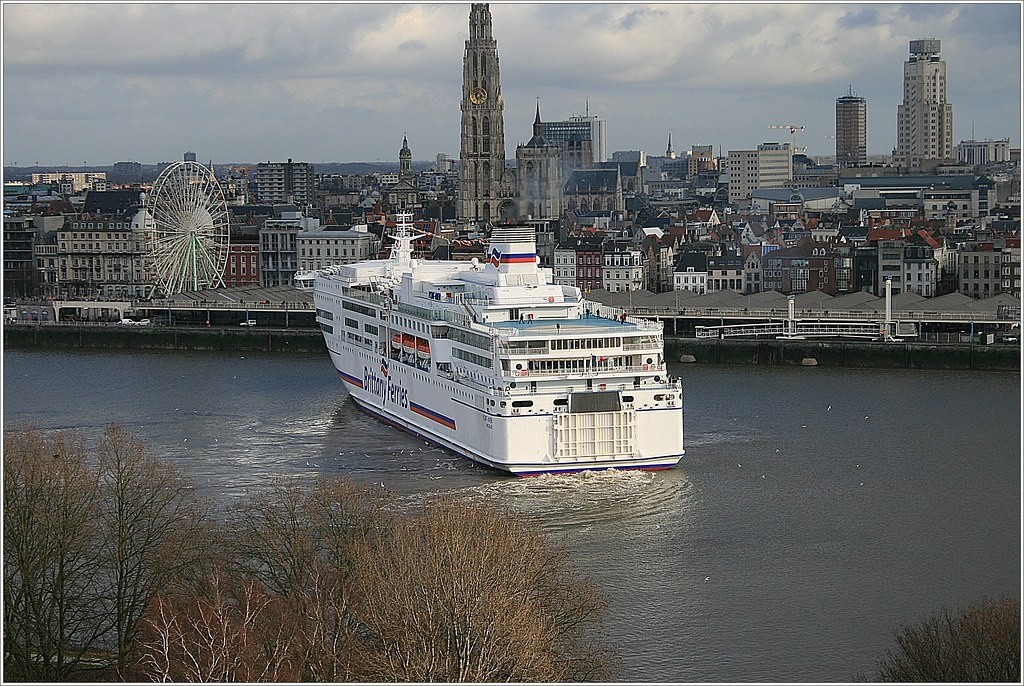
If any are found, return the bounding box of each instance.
[313,228,685,476]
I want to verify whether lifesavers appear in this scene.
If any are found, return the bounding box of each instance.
[647,358,653,364]
[510,382,516,388]
[660,380,664,384]
[506,386,510,391]
[654,375,660,382]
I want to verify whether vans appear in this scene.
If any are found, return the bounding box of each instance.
[119,318,150,327]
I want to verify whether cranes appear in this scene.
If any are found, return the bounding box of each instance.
[766,124,806,145]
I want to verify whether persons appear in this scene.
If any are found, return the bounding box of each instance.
[556,322,561,334]
[519,312,526,324]
[497,385,511,398]
[666,372,682,384]
[596,308,601,319]
[470,256,479,271]
[620,310,628,325]
[586,307,590,319]
[527,313,534,324]
